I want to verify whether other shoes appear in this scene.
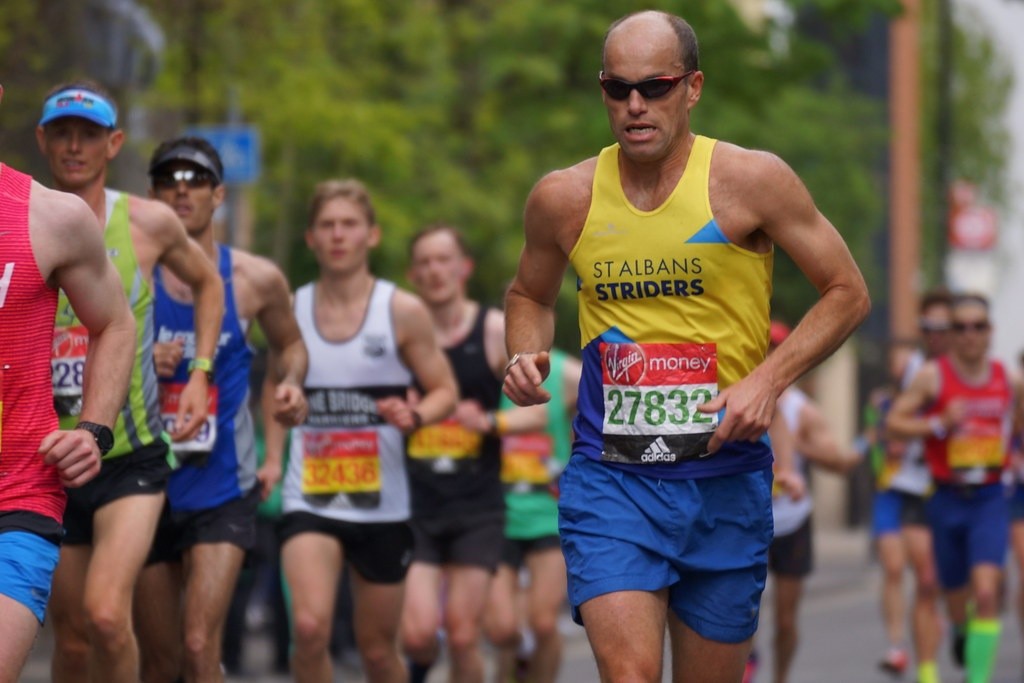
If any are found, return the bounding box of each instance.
[882,648,908,672]
[952,625,969,666]
[741,651,758,683]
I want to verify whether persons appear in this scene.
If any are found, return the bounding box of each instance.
[742,322,859,683]
[863,294,1024,683]
[133,136,309,683]
[0,85,135,683]
[503,10,871,683]
[481,351,582,683]
[257,180,458,683]
[399,223,509,683]
[35,80,224,683]
[224,328,356,674]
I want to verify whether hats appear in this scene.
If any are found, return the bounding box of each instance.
[39,88,118,132]
[148,143,222,183]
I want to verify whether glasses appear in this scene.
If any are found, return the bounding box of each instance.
[921,322,951,334]
[598,70,694,100]
[152,163,212,187]
[950,321,989,333]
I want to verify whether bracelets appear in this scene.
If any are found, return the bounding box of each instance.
[489,411,505,432]
[932,417,946,439]
[187,357,213,378]
[505,353,520,370]
[410,409,422,428]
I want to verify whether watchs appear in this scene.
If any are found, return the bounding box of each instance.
[75,422,113,457]
